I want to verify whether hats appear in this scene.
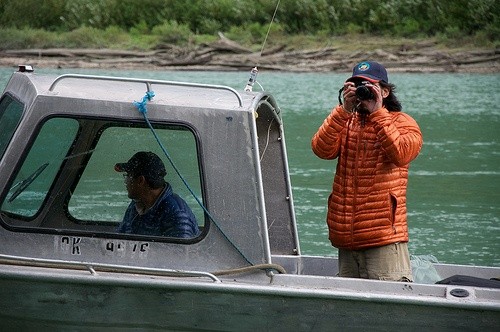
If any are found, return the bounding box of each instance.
[345,61,388,84]
[114,151,166,178]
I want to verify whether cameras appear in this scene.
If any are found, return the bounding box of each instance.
[352,81,374,100]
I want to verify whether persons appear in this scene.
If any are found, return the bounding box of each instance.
[114,151,200,239]
[311,62,423,282]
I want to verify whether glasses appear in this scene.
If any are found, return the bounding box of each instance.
[122,172,131,179]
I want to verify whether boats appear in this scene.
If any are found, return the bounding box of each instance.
[0,65,500,332]
